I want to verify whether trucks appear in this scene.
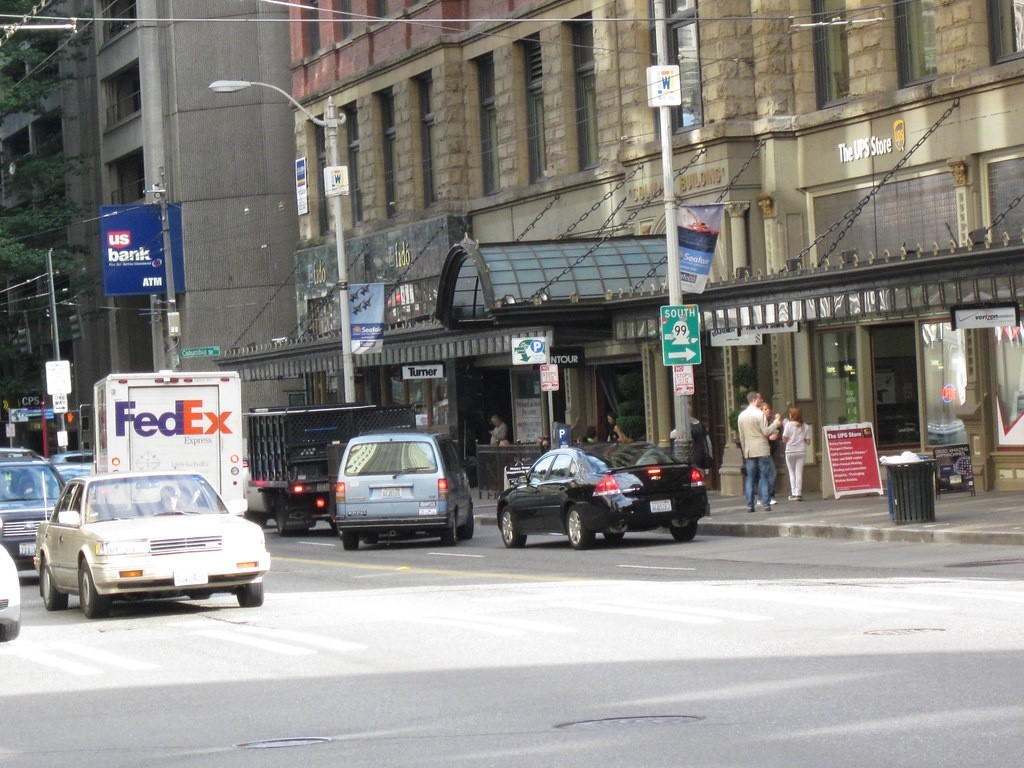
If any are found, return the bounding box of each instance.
[241,401,417,537]
[78,370,248,519]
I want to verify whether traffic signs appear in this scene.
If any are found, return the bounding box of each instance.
[659,305,701,367]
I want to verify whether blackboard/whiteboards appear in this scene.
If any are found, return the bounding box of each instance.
[933,443,974,494]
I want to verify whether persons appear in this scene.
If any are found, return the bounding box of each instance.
[605,412,624,442]
[737,391,782,512]
[160,485,178,511]
[782,406,810,501]
[488,414,508,444]
[669,398,713,474]
[582,425,597,443]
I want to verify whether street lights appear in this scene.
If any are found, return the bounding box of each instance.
[207,80,355,402]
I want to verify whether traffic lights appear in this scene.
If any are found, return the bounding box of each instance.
[64,410,80,426]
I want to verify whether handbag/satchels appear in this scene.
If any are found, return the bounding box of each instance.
[704,454,713,468]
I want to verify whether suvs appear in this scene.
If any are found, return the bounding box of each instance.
[335,431,474,551]
[1,452,67,573]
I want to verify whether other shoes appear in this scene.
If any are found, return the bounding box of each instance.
[765,508,770,511]
[747,508,754,513]
[770,499,777,506]
[757,499,765,506]
[787,493,801,502]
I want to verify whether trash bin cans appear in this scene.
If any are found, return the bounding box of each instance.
[882,456,938,525]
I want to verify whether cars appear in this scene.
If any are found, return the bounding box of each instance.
[34,469,271,619]
[0,542,22,643]
[1,447,94,487]
[496,439,709,551]
